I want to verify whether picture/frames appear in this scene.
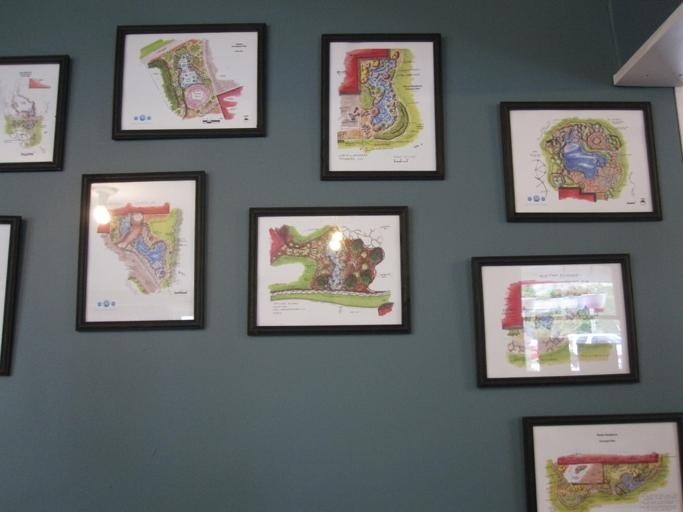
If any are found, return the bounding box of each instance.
[74,169,208,331]
[1,215,28,378]
[522,411,683,510]
[246,205,411,337]
[111,24,267,141]
[0,55,72,172]
[318,32,443,182]
[498,102,660,223]
[473,252,642,387]
